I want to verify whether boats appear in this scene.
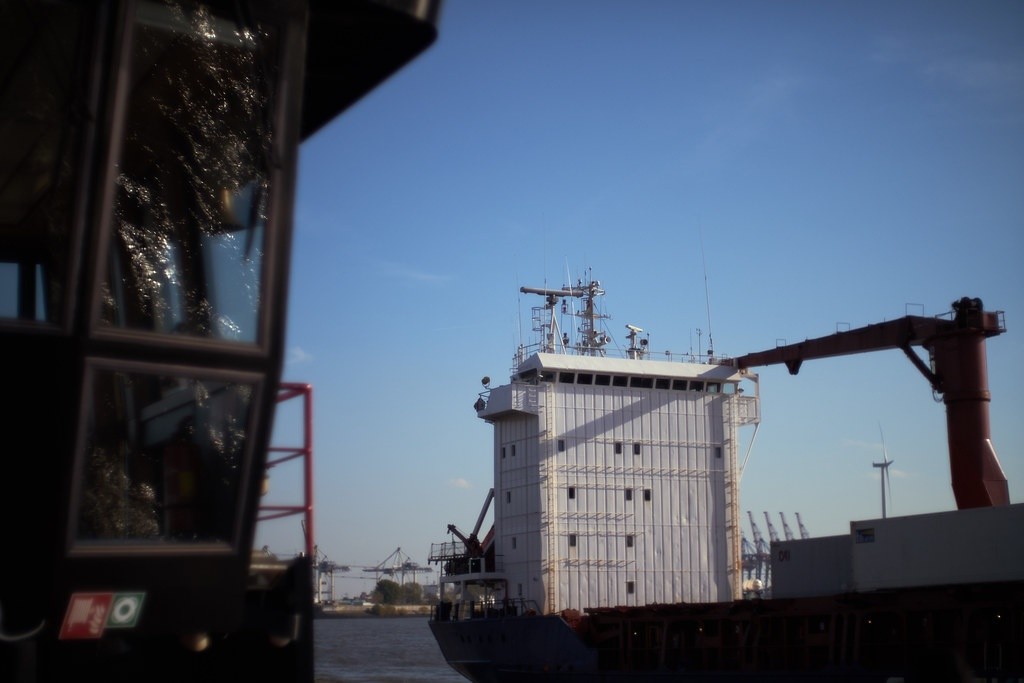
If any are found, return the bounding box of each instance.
[426,261,1023,683]
